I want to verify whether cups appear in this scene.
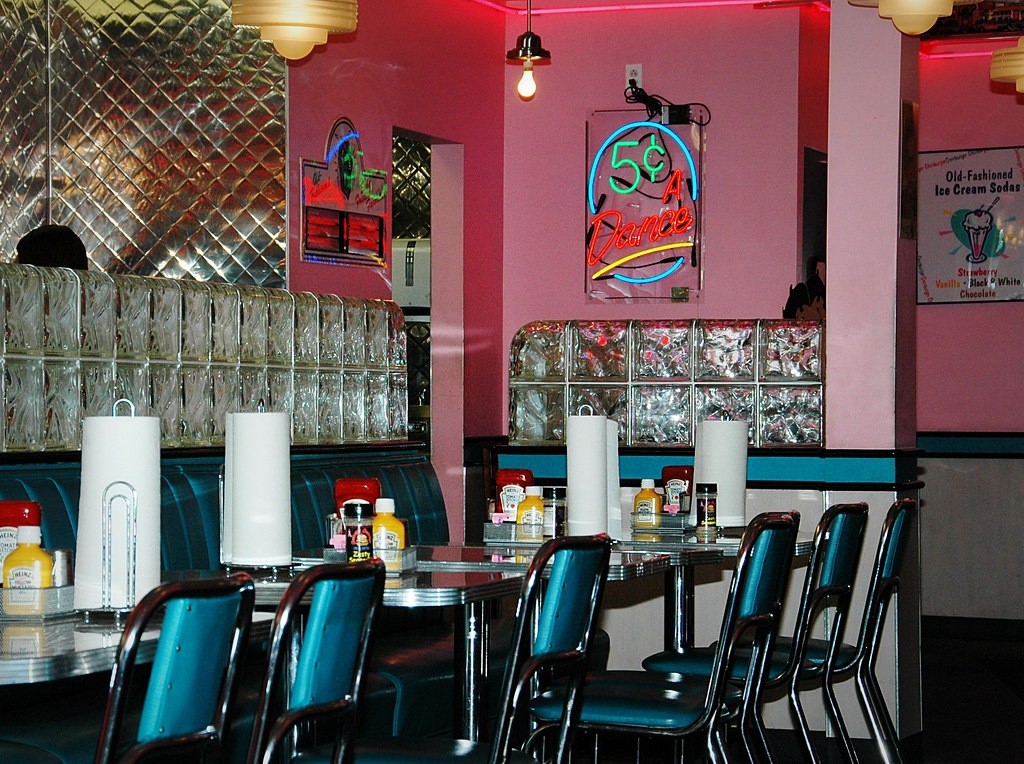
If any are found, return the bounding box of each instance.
[42,547,75,589]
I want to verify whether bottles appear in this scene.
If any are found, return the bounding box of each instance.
[495,468,533,524]
[372,498,404,570]
[634,479,661,527]
[0,500,42,615]
[542,488,566,540]
[344,503,373,562]
[662,466,694,514]
[696,483,717,544]
[516,486,544,540]
[4,525,54,614]
[335,478,381,535]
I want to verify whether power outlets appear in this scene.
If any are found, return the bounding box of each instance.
[625,64,643,92]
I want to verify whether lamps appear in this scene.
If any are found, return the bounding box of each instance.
[73,397,162,629]
[230,1,358,60]
[506,1,550,98]
[564,405,623,543]
[692,420,749,538]
[990,38,1023,92]
[219,399,291,584]
[847,1,953,36]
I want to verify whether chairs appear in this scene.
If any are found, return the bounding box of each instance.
[1,500,917,763]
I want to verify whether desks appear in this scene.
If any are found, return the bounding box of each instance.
[607,530,834,558]
[414,550,722,656]
[237,574,546,741]
[1,608,278,687]
